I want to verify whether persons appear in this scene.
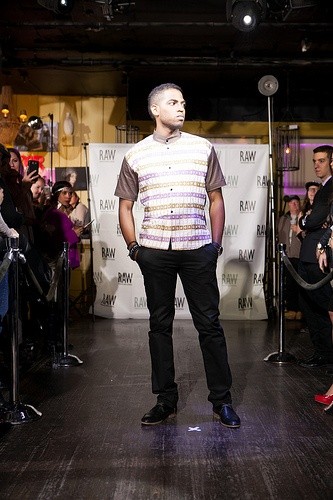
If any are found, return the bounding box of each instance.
[114,83,241,428]
[278,145,333,411]
[0,144,90,415]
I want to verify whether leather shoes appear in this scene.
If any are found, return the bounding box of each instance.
[212,403,240,428]
[140,394,177,425]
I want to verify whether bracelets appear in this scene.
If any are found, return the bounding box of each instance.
[320,248,326,254]
[127,241,139,261]
[213,241,223,255]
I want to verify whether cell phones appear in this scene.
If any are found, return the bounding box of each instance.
[27,160,39,181]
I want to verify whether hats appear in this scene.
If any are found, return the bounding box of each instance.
[304,182,320,190]
[288,195,300,202]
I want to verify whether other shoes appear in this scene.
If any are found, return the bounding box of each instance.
[299,354,333,368]
[287,310,296,320]
[295,311,302,319]
[313,393,333,405]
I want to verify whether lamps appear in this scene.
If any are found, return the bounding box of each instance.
[275,124,300,171]
[2,104,10,118]
[232,2,261,33]
[19,110,28,123]
